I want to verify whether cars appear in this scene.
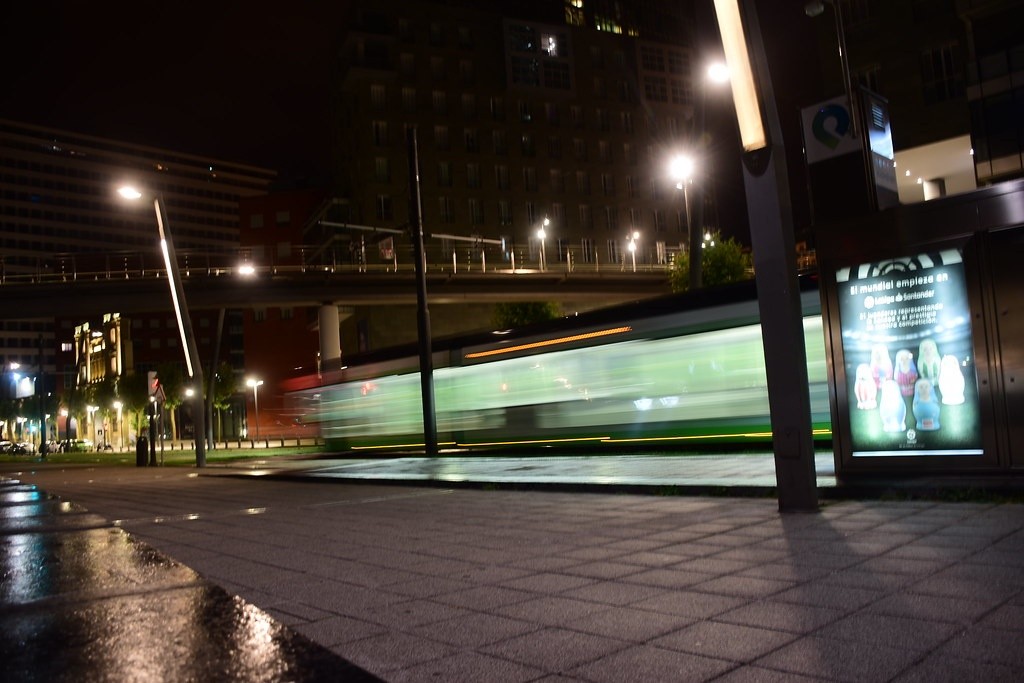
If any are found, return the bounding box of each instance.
[0,437,94,454]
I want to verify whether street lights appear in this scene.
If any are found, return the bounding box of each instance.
[117,185,205,467]
[668,153,695,246]
[538,217,550,269]
[628,232,641,274]
